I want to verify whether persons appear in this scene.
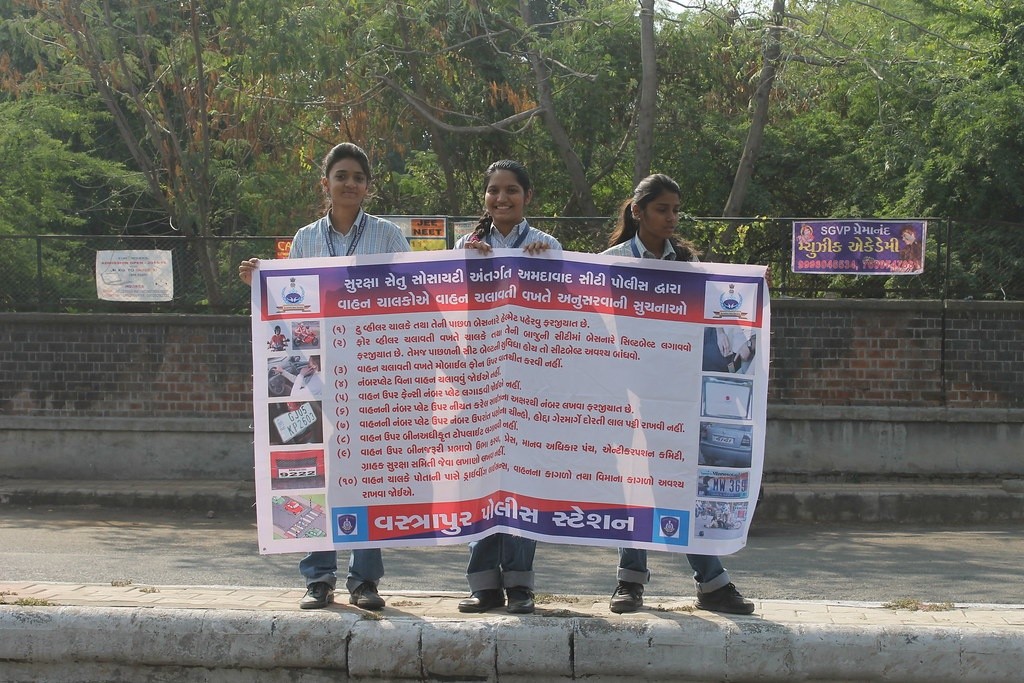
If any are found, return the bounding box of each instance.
[452,160,562,614]
[267,325,288,348]
[716,327,756,361]
[295,323,312,343]
[697,500,731,529]
[272,355,322,396]
[900,226,922,272]
[598,173,771,614]
[239,143,414,609]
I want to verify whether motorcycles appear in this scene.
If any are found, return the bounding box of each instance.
[266,338,290,351]
[293,331,319,347]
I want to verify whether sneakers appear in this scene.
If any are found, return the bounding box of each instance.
[349,582,385,607]
[458,589,505,612]
[506,585,535,613]
[695,582,754,615]
[609,580,644,612]
[300,582,334,608]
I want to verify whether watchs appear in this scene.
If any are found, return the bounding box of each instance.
[746,339,755,355]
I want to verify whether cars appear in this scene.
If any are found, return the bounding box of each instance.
[700,423,751,467]
